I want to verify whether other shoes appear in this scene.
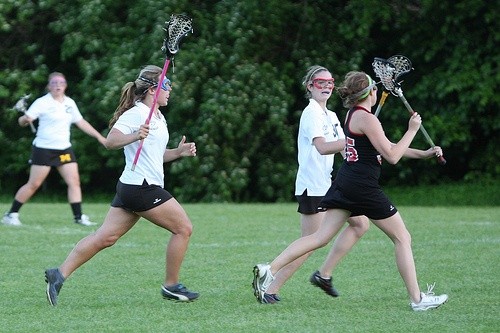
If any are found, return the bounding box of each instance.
[1,212,23,225]
[74,214,97,226]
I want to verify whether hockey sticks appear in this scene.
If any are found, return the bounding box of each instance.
[130,11,194,172]
[13,98,38,134]
[388,54,440,160]
[371,57,397,117]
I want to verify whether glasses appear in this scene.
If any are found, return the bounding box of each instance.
[309,78,335,90]
[48,79,66,87]
[139,75,172,91]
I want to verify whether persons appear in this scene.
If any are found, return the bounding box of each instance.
[1,72,107,225]
[256,66,370,304]
[45,65,201,308]
[252,72,449,312]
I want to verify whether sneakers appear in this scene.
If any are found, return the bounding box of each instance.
[409,292,448,312]
[260,293,282,304]
[44,268,64,306]
[253,263,274,304]
[309,270,339,299]
[162,283,200,303]
